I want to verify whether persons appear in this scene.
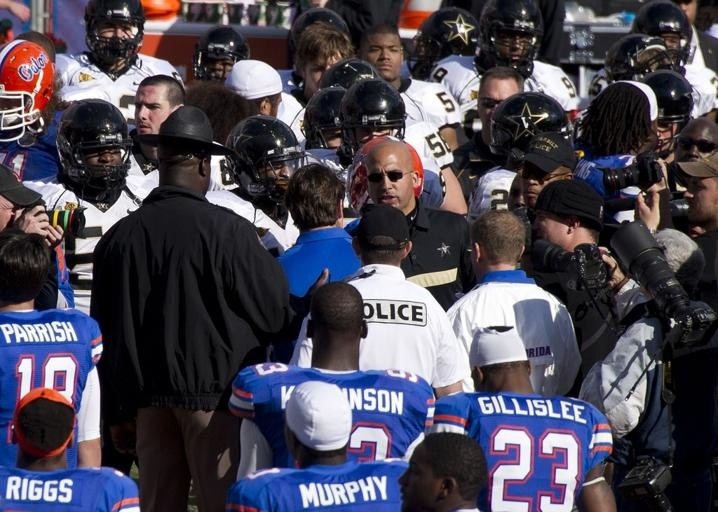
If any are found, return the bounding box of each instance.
[0,0,718,511]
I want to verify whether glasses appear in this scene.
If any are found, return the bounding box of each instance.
[522,168,569,184]
[679,139,716,152]
[365,171,404,183]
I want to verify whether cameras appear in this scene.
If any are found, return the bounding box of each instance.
[597,157,662,191]
[619,464,673,511]
[22,206,86,236]
[532,240,608,291]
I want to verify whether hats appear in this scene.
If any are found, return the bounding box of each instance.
[357,205,409,250]
[535,180,604,232]
[519,132,578,174]
[674,148,718,187]
[222,61,285,100]
[0,164,43,206]
[133,106,234,154]
[285,380,353,450]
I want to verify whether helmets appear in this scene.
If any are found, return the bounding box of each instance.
[85,2,146,73]
[56,98,133,192]
[0,39,54,142]
[490,92,573,157]
[604,1,695,156]
[477,0,545,75]
[409,6,480,79]
[300,56,407,163]
[224,114,309,202]
[288,8,351,60]
[192,26,252,79]
